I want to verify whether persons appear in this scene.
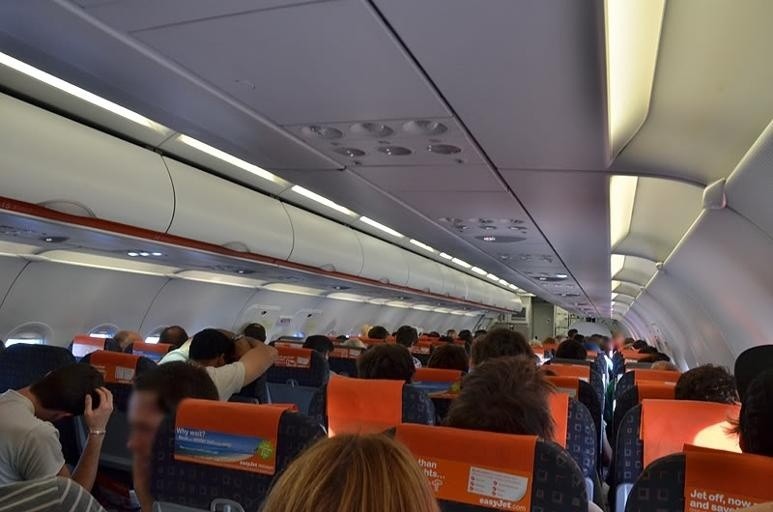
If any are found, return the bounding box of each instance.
[1,322,773,510]
